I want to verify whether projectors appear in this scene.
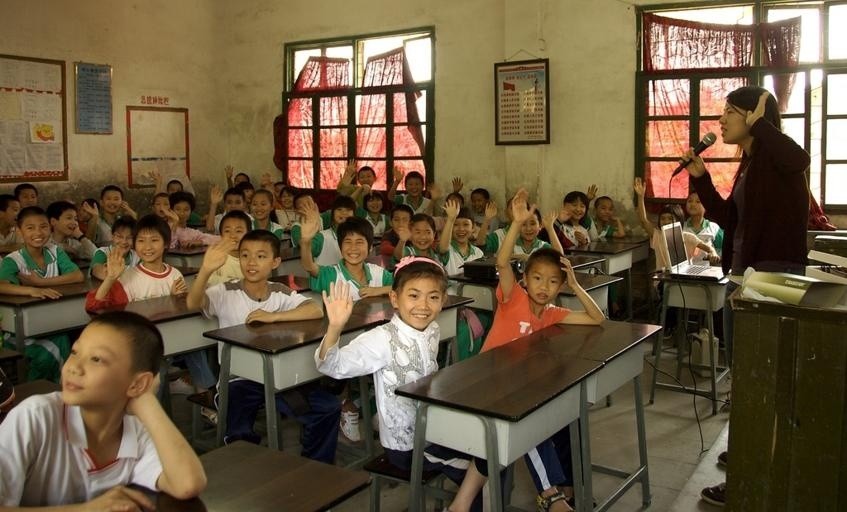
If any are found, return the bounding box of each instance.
[464,256,526,281]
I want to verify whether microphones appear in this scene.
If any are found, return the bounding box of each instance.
[672,132,716,177]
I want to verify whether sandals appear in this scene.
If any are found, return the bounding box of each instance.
[536,491,577,512]
[200,405,219,425]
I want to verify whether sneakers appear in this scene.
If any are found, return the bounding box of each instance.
[718,451,728,467]
[167,365,182,382]
[700,482,727,507]
[168,376,209,395]
[339,398,361,442]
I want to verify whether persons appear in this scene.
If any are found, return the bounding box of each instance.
[679,84,810,507]
[1,158,722,443]
[312,254,506,512]
[478,187,608,512]
[0,310,208,512]
[185,228,341,465]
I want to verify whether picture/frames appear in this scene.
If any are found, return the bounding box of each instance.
[494,59,550,145]
[73,61,114,135]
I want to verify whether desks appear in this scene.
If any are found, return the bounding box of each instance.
[1,225,732,512]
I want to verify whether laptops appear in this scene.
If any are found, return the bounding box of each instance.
[661,222,725,281]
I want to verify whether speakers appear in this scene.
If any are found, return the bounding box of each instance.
[692,329,719,377]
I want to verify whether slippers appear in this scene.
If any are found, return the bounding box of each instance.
[185,390,219,410]
